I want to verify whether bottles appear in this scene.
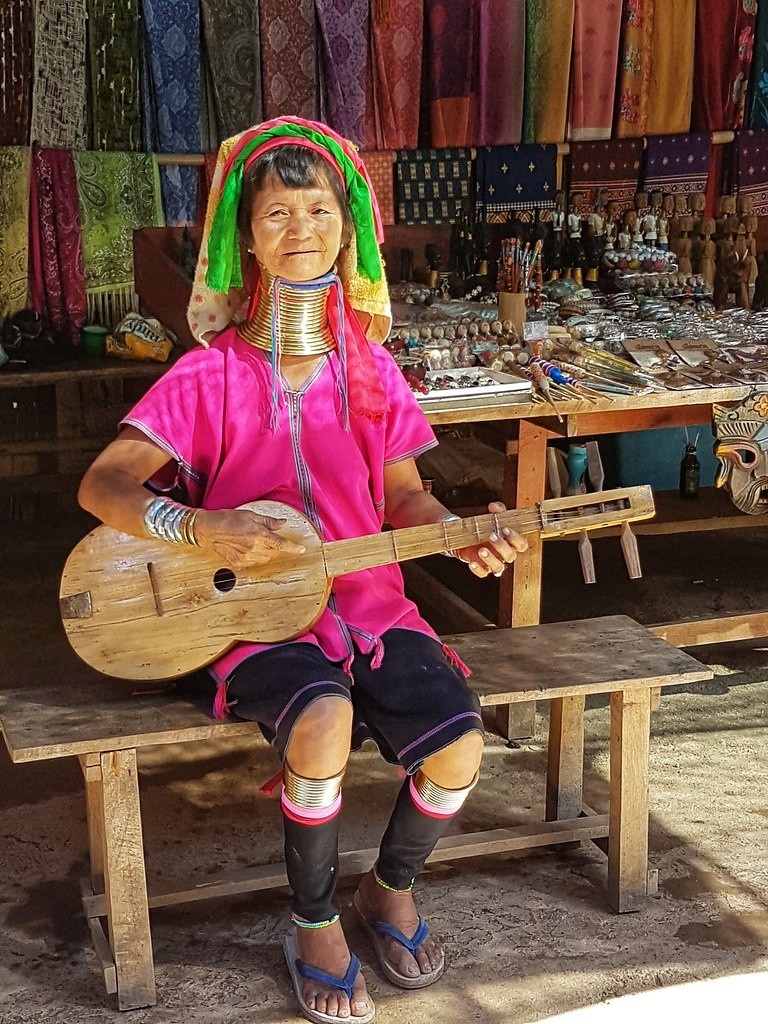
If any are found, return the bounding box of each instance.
[680,447,701,499]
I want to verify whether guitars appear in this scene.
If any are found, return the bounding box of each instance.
[58,440,658,684]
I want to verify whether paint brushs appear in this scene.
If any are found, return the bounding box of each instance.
[501,237,542,293]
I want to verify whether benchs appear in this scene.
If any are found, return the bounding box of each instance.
[0,613,713,1012]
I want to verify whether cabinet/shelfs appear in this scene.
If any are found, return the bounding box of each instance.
[0,346,175,454]
[418,383,768,741]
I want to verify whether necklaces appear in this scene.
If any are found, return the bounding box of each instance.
[238,259,338,355]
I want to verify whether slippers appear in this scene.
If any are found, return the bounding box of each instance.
[283,922,377,1024]
[350,887,446,990]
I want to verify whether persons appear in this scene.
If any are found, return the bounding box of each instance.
[78,117,528,1024]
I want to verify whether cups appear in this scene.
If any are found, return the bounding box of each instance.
[83,325,110,356]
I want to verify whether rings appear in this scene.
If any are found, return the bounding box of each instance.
[493,564,506,579]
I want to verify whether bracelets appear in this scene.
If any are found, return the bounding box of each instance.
[434,514,470,563]
[144,497,205,548]
